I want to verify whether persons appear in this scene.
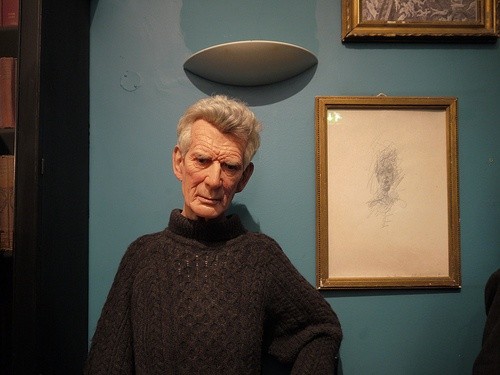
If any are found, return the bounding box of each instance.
[81,95,343,375]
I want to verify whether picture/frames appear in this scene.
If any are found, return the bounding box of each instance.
[314,92,464,293]
[340,0,500,43]
